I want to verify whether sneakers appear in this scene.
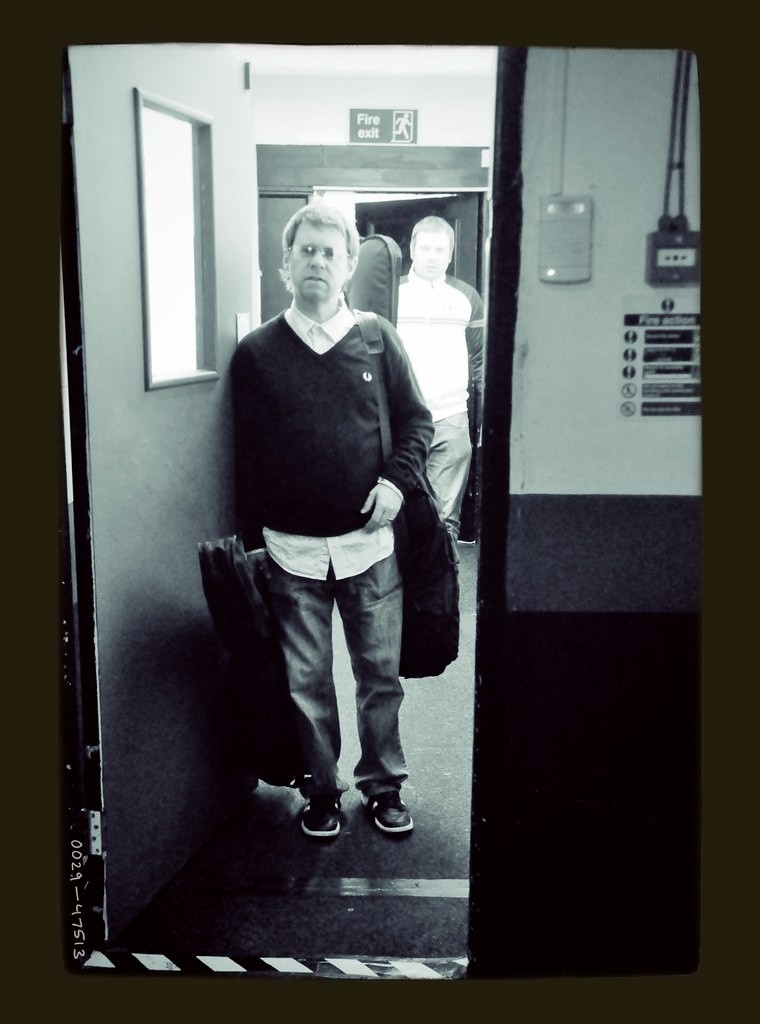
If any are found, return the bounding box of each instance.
[301,792,341,837]
[360,787,413,833]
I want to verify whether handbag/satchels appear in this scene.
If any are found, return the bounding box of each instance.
[194,538,304,786]
[347,236,459,678]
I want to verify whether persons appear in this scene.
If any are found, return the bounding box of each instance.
[389,217,484,565]
[220,202,436,841]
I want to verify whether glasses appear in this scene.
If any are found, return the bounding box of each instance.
[288,244,351,261]
